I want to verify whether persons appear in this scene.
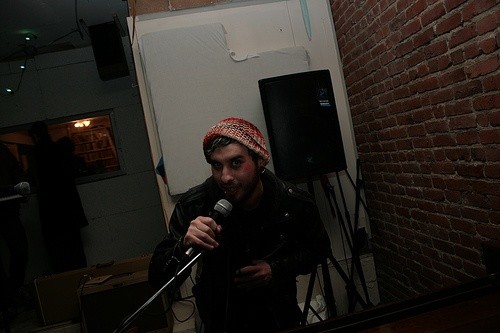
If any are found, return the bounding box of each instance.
[0,142,32,314]
[148,117,333,333]
[26,119,92,273]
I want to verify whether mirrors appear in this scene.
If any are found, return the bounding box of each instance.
[0,107,128,203]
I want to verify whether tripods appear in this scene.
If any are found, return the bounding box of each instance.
[301,177,367,328]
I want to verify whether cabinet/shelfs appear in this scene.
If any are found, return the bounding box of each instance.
[66,125,114,166]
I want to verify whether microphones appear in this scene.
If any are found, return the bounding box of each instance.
[0,181,31,196]
[178,198,233,266]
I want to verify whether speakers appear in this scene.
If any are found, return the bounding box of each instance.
[78,269,174,333]
[258,69,348,181]
[87,21,131,82]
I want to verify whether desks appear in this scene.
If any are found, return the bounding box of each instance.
[76,269,173,333]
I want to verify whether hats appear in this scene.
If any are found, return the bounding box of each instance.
[203,117,270,165]
